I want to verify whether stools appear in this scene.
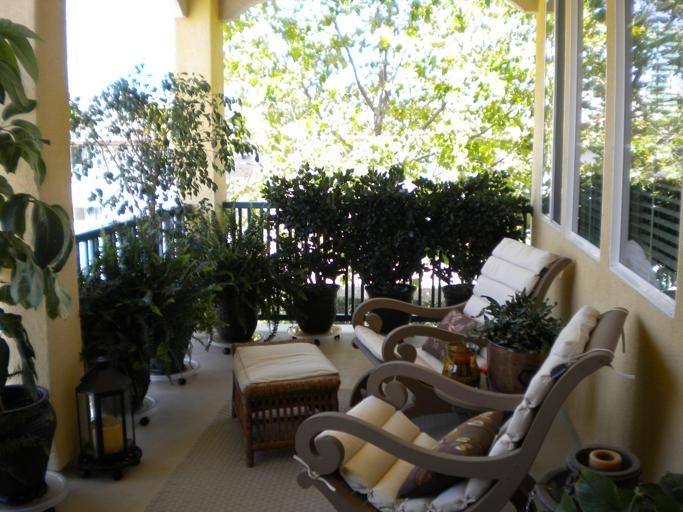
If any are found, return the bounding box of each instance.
[225,334,345,472]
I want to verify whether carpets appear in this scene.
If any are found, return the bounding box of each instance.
[143,387,377,512]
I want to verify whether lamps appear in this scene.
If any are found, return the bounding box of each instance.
[72,356,143,482]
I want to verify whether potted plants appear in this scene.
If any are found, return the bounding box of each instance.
[0,16,74,507]
